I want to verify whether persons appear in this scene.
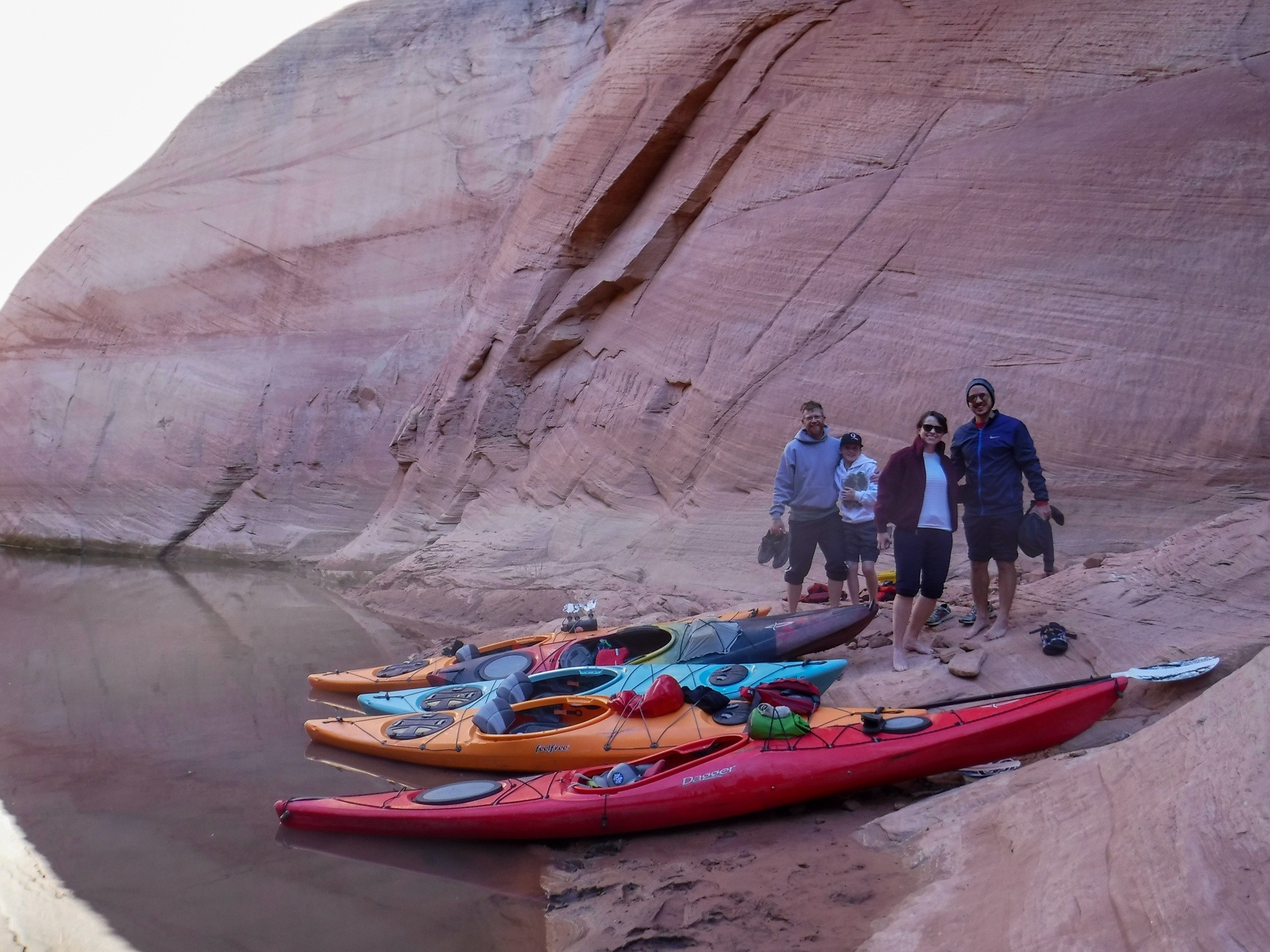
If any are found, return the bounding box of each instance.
[874,378,1052,672]
[768,402,882,617]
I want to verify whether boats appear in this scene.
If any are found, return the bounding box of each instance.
[305,695,932,773]
[357,656,849,716]
[275,674,1135,843]
[307,602,885,694]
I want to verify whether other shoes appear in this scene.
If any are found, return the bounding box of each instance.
[842,471,868,510]
[758,528,792,568]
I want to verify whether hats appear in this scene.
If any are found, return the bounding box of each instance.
[604,763,642,787]
[966,378,995,408]
[840,433,863,447]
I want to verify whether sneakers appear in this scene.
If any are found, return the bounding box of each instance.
[924,603,953,625]
[958,601,996,624]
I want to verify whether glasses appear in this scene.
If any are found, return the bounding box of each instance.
[967,393,990,403]
[922,423,944,434]
[804,414,822,422]
